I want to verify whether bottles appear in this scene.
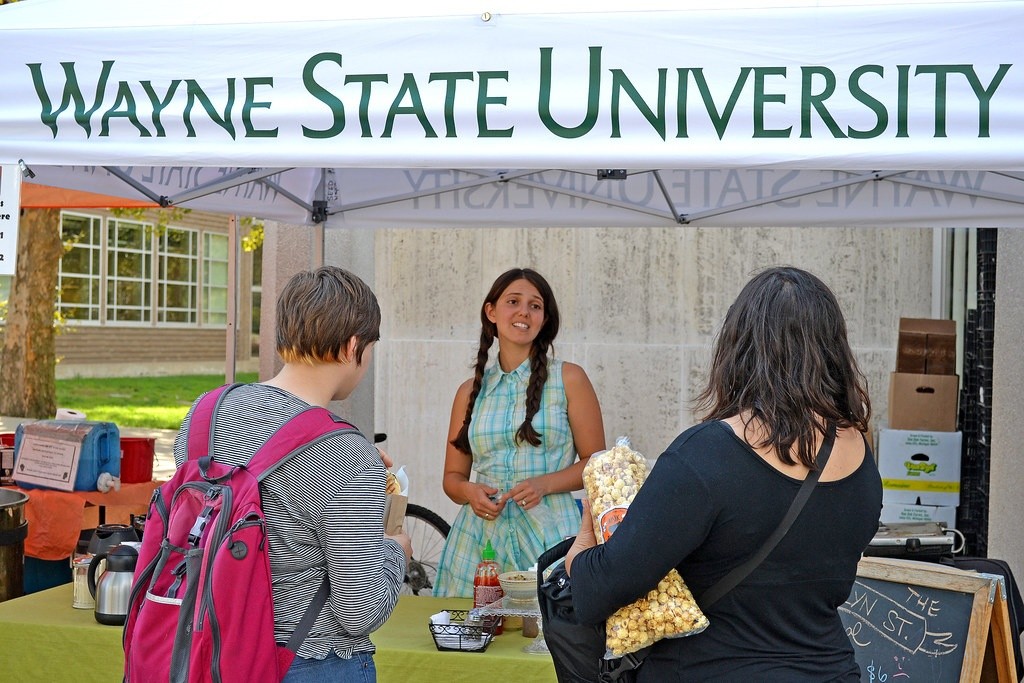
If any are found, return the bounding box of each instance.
[473,539,504,635]
[72,553,102,609]
[464,608,483,644]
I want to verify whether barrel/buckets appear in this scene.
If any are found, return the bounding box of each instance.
[119,437,157,484]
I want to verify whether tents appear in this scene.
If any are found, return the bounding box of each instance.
[1,0,1024,388]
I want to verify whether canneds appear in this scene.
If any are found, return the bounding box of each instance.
[0,434,18,487]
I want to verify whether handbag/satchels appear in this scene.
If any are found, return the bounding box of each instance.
[536,537,627,683]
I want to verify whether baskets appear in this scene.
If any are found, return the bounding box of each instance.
[429,610,501,652]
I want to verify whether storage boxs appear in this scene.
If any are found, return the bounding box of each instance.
[897,318,956,377]
[889,373,958,432]
[877,427,961,506]
[879,501,956,546]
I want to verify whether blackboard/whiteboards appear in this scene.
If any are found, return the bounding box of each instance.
[837,557,1018,683]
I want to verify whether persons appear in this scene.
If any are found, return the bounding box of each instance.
[564,264,883,683]
[431,267,607,597]
[172,267,413,682]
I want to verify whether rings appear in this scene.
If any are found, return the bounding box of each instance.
[485,513,489,518]
[522,499,526,506]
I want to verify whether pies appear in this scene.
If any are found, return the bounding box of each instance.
[386,473,401,494]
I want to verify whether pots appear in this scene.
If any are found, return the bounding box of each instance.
[0,488,29,602]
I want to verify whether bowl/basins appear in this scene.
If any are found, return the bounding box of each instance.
[497,570,537,603]
[0,432,16,446]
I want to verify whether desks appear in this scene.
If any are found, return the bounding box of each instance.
[0,578,557,683]
[1,482,166,591]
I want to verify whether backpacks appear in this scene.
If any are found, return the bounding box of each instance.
[123,382,367,683]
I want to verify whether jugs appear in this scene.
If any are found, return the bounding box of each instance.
[88,546,139,626]
